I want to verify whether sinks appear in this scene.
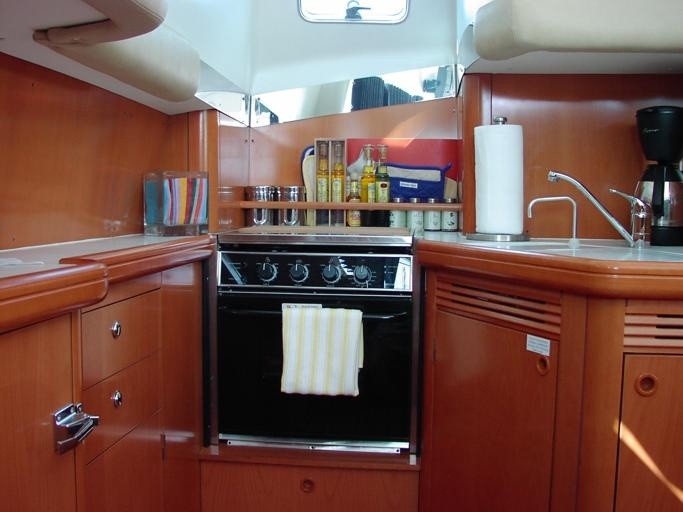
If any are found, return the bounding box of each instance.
[461,240,611,252]
[524,244,683,263]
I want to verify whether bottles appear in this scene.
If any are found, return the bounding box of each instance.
[274,184,307,226]
[359,145,376,225]
[244,185,274,227]
[440,197,457,230]
[424,198,439,231]
[316,143,327,224]
[374,142,390,226]
[388,196,404,230]
[406,198,423,235]
[346,173,361,226]
[331,142,344,223]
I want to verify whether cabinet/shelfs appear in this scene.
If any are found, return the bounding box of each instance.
[576,296,683,512]
[81,261,204,512]
[418,268,587,511]
[0,309,73,512]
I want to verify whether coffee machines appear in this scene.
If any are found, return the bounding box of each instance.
[628,105,683,247]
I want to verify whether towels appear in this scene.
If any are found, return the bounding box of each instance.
[279,306,364,398]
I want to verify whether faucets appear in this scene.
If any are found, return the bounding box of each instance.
[547,170,652,250]
[527,196,577,237]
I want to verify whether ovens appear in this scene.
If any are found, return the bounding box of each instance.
[212,233,416,458]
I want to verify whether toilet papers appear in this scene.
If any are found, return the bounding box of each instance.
[474,124,524,234]
